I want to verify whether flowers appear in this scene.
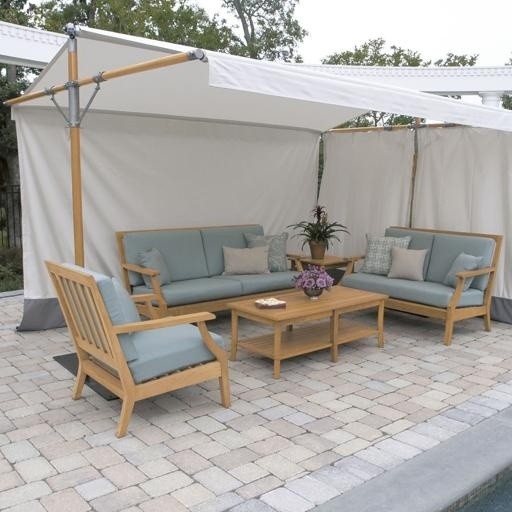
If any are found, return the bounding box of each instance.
[288,264,335,291]
[285,205,350,251]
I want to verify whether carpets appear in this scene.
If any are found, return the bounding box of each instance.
[52,352,121,401]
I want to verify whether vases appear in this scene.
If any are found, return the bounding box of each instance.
[303,288,324,296]
[308,240,328,259]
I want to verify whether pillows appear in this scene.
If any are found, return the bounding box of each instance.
[442,252,482,292]
[111,275,141,335]
[358,232,430,281]
[136,248,171,288]
[220,231,289,276]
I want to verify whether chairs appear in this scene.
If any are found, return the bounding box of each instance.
[44,259,232,438]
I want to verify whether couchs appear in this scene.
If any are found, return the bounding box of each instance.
[115,225,303,319]
[341,226,504,346]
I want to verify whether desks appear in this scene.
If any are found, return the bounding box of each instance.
[294,256,364,286]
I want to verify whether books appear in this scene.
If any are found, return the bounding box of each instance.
[255,296,288,310]
